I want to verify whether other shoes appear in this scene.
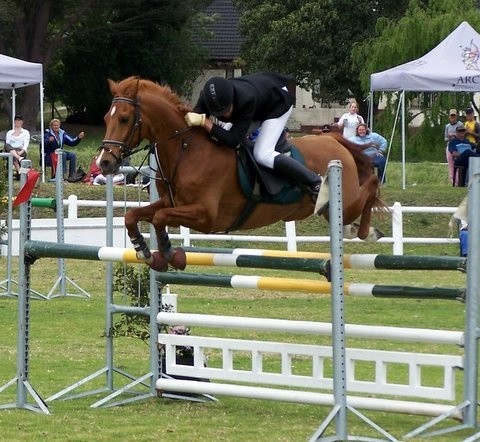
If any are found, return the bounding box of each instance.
[18,156,25,162]
[68,177,74,181]
[49,177,57,181]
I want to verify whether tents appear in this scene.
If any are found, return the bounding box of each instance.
[1,54,45,181]
[369,21,479,189]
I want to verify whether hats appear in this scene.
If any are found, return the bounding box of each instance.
[465,107,474,114]
[14,114,24,120]
[456,126,465,131]
[202,77,234,116]
[449,108,458,116]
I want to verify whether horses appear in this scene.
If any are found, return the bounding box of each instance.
[95,74,400,272]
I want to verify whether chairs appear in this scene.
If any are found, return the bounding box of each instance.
[40,142,55,178]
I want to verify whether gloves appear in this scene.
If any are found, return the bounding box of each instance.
[185,112,205,127]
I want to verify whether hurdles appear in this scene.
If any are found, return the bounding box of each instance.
[0,159,401,442]
[88,151,480,439]
[0,149,92,300]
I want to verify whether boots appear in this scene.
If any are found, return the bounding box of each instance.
[273,153,329,216]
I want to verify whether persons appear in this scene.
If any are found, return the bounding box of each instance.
[334,101,365,140]
[4,114,30,179]
[347,124,387,184]
[39,119,84,182]
[444,109,463,186]
[448,125,480,187]
[462,107,480,153]
[184,71,329,216]
